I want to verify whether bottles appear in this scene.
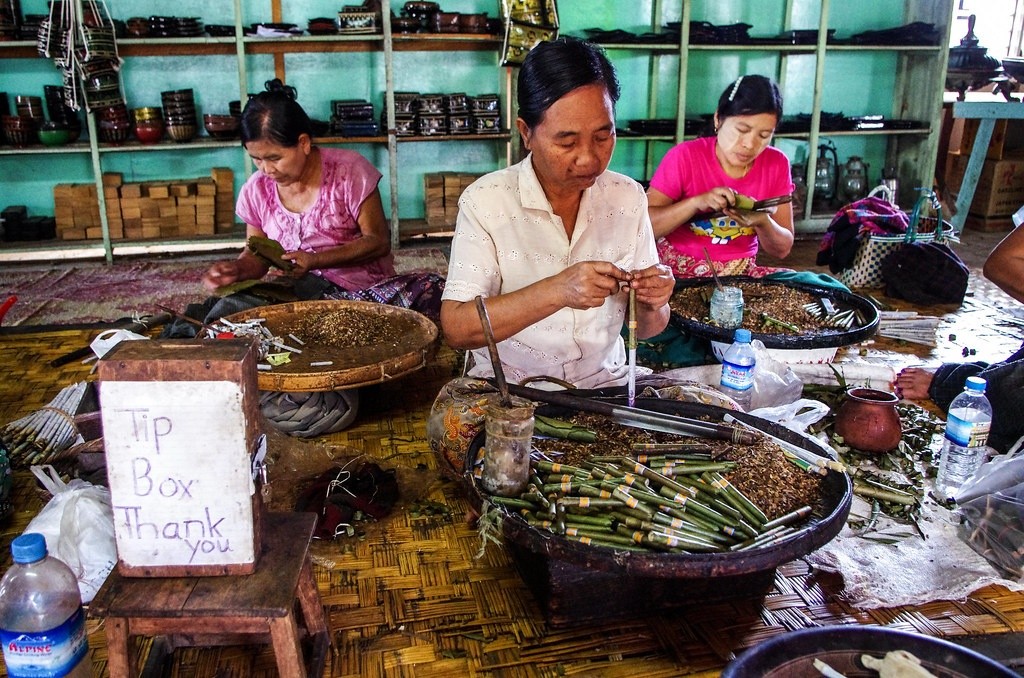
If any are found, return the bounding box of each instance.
[719,329,757,414]
[0,533,94,678]
[933,376,992,503]
[834,388,902,455]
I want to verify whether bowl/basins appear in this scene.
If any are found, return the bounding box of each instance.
[127,17,148,38]
[723,625,1024,678]
[0,0,46,41]
[100,88,240,146]
[0,85,83,149]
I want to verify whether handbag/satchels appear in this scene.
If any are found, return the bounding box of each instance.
[885,191,969,308]
[10,465,118,604]
[37,0,124,111]
[839,184,954,288]
[498,0,560,68]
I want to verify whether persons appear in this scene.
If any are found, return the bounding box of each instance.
[897,222,1024,454]
[203,78,446,333]
[426,36,744,483]
[645,75,851,293]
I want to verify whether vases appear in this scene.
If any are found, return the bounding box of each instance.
[835,387,904,453]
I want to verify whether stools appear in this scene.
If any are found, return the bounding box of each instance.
[88,510,330,678]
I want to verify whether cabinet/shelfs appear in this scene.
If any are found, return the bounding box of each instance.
[0,0,956,266]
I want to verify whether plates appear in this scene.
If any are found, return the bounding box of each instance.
[306,17,338,35]
[243,22,303,36]
[582,19,939,136]
[149,15,236,38]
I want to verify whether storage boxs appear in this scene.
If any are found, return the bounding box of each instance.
[512,544,775,631]
[328,91,502,136]
[940,107,1024,234]
[423,170,482,227]
[54,167,236,241]
[97,340,265,579]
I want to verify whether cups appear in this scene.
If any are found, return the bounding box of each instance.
[482,397,534,497]
[710,286,743,329]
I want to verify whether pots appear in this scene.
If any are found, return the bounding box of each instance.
[391,0,502,36]
